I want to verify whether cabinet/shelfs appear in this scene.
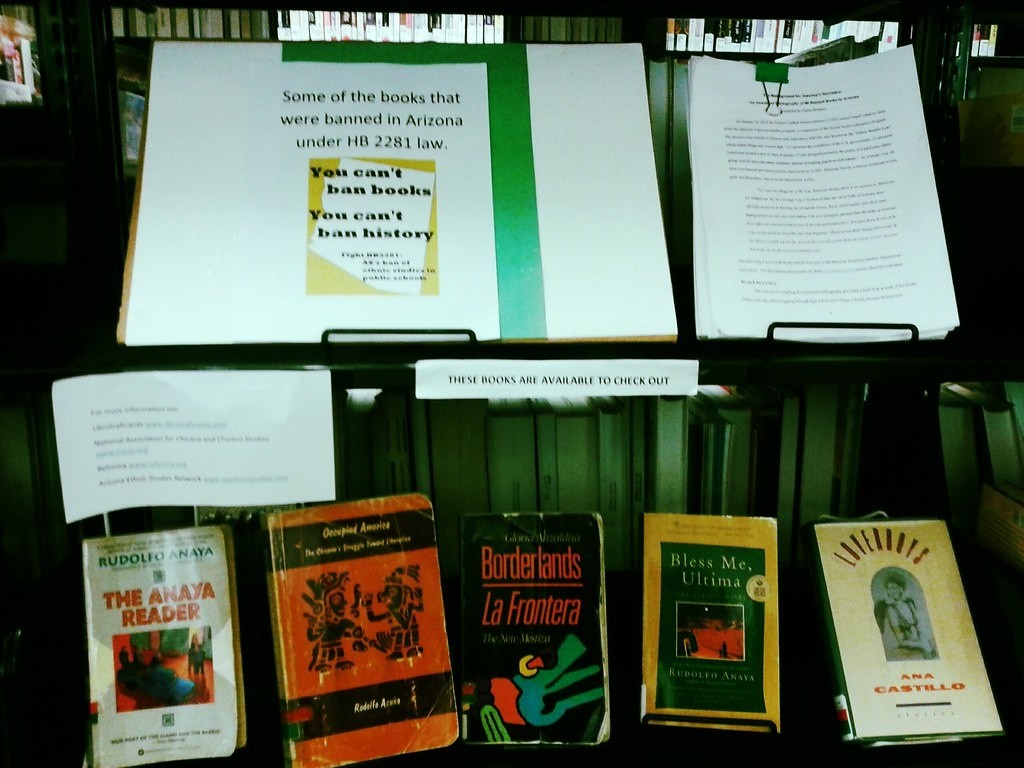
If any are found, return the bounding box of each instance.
[0,0,1024,768]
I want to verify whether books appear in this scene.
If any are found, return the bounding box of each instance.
[82,525,246,768]
[460,512,610,746]
[813,519,1005,747]
[260,494,459,768]
[641,513,781,735]
